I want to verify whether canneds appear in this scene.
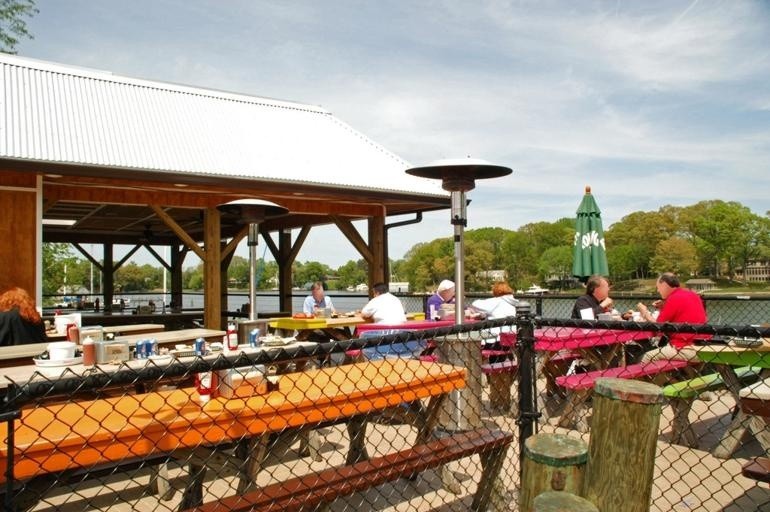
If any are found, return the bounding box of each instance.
[195,338,205,355]
[136,338,158,359]
[250,331,258,348]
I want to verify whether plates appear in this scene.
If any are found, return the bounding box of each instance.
[732,336,764,347]
[31,353,85,367]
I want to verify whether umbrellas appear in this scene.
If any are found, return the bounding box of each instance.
[569,186,611,286]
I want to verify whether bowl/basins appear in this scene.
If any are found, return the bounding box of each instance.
[46,340,77,359]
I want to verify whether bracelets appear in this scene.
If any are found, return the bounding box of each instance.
[642,309,649,318]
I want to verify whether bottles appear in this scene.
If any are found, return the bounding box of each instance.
[82,336,96,368]
[227,323,239,350]
[198,371,212,403]
[69,323,79,346]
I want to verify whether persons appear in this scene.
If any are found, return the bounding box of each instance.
[355,281,407,327]
[635,272,713,385]
[425,279,462,322]
[469,280,521,364]
[304,282,351,367]
[0,287,48,347]
[569,276,641,374]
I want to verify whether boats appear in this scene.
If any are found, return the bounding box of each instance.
[524,286,550,294]
[346,286,354,292]
[96,296,131,308]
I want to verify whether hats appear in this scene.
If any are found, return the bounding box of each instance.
[438,280,454,292]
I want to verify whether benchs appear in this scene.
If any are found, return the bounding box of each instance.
[181,427,514,512]
[268,314,770,460]
